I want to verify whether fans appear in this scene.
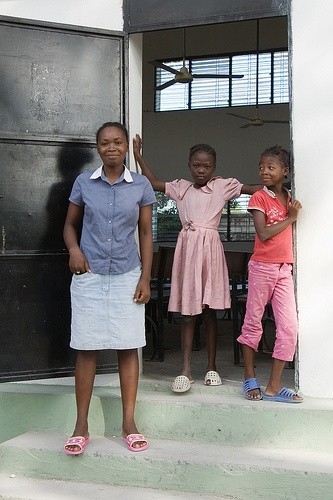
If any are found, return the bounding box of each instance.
[225,20,290,129]
[148,28,245,90]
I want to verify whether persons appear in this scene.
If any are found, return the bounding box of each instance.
[132,134,292,393]
[236,145,303,403]
[64,122,157,454]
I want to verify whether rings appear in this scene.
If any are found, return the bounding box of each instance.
[76,271,80,273]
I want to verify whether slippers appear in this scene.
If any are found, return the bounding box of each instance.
[122,432,148,451]
[203,371,223,385]
[172,375,195,392]
[263,386,303,402]
[65,434,90,453]
[242,377,264,401]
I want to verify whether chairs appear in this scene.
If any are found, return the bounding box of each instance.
[143,242,296,371]
